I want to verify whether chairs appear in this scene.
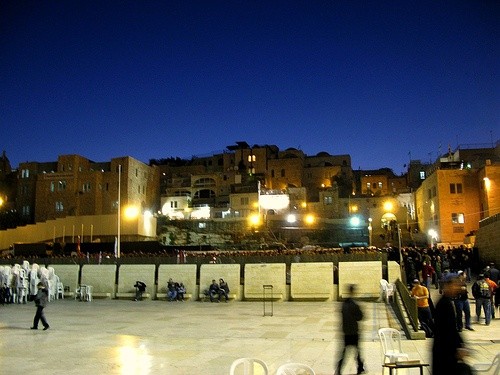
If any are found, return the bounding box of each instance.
[378,328,408,375]
[75,284,92,302]
[380,278,394,305]
[472,352,500,375]
[229,357,268,375]
[0,261,64,303]
[275,363,316,375]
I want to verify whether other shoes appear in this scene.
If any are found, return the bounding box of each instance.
[31,328,38,329]
[466,327,473,331]
[43,325,49,331]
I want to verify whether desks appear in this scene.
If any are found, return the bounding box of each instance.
[382,364,430,375]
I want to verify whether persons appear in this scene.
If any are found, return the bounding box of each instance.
[133,281,147,302]
[216,278,230,302]
[335,284,365,375]
[177,281,186,302]
[409,260,500,375]
[0,244,478,288]
[167,279,177,302]
[0,283,11,305]
[28,282,51,330]
[204,280,219,302]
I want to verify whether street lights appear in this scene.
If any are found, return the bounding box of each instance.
[428,229,437,250]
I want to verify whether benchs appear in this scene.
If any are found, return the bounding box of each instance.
[340,293,381,302]
[91,293,111,298]
[157,293,192,302]
[116,292,150,300]
[291,294,330,302]
[64,292,75,298]
[199,294,237,303]
[244,294,282,302]
